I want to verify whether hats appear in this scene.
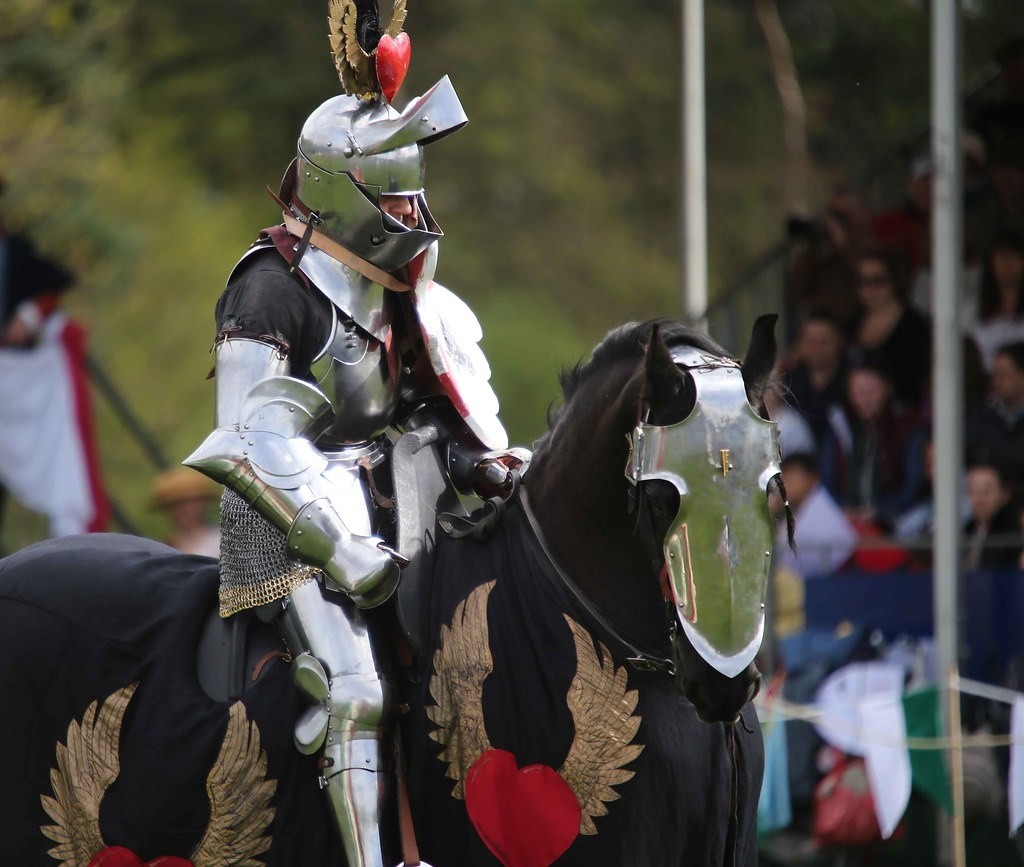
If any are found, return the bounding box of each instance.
[0,230,74,336]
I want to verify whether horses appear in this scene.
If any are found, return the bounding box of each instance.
[0,307,808,867]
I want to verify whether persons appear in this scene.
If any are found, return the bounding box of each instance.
[149,472,221,560]
[0,231,109,563]
[754,0,1024,867]
[181,93,531,867]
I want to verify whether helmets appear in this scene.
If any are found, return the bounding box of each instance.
[273,1,468,274]
